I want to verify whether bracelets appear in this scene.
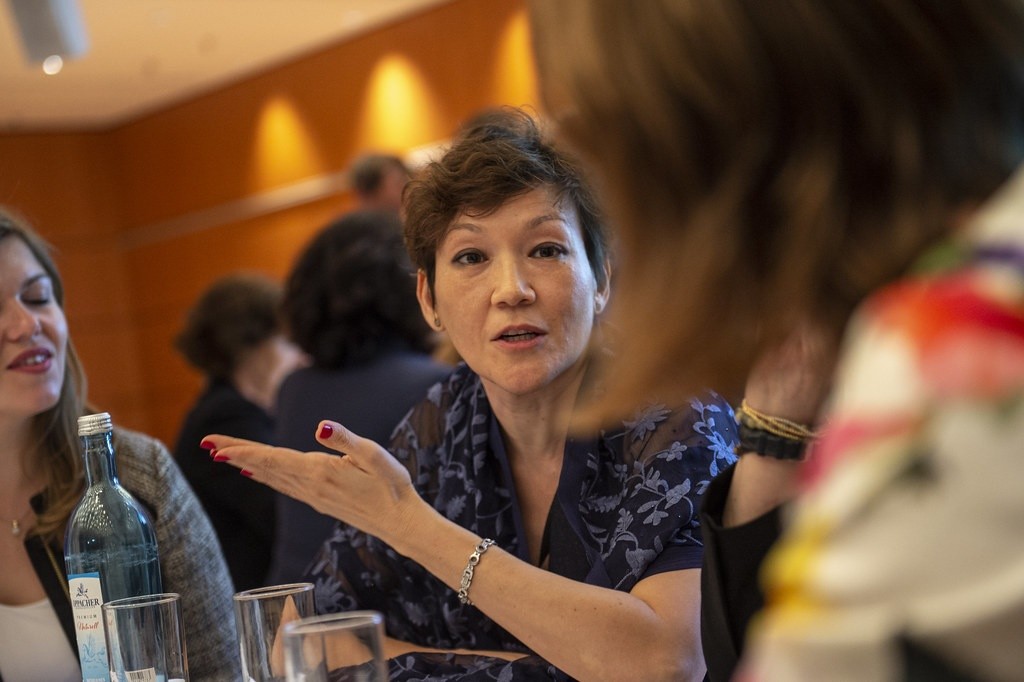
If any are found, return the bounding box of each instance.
[457,538,498,606]
[733,400,813,462]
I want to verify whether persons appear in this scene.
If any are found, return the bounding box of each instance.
[200,107,740,682]
[0,211,244,682]
[168,152,459,682]
[528,0,1023,682]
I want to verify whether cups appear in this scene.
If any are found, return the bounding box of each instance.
[280,609,390,682]
[101,593,190,682]
[232,583,315,682]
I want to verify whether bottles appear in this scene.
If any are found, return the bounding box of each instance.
[65,412,168,682]
